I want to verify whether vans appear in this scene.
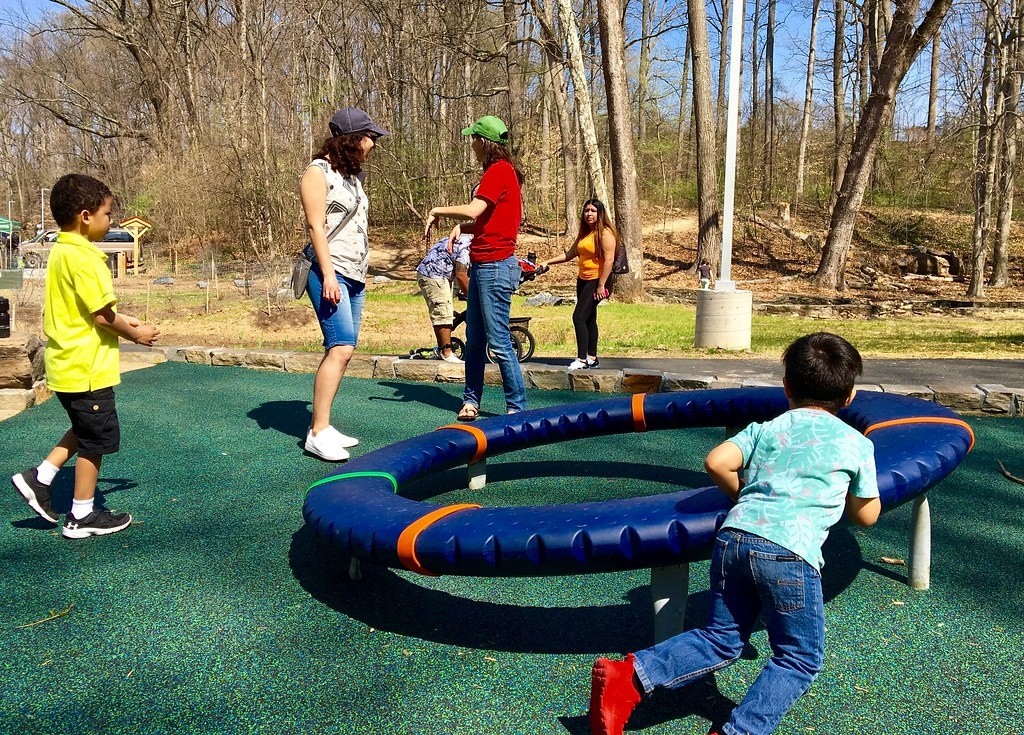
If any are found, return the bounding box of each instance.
[15,228,146,270]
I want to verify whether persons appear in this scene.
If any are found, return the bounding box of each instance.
[11,173,160,539]
[416,234,472,364]
[590,332,882,735]
[300,106,389,459]
[424,116,526,420]
[540,199,621,370]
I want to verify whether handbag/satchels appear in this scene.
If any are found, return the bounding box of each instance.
[290,250,312,299]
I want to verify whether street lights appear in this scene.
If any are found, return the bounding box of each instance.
[9,201,15,272]
[41,188,51,231]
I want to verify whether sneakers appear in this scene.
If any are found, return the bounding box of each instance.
[11,467,59,523]
[62,504,132,538]
[304,429,350,460]
[307,424,358,447]
[589,653,640,735]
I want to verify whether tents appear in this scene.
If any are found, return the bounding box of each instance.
[0,216,22,270]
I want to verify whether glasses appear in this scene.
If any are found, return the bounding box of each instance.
[361,133,377,148]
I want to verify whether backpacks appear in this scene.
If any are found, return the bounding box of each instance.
[612,240,629,274]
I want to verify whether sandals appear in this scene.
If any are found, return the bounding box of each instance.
[459,405,479,420]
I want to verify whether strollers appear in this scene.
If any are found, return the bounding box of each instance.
[435,253,550,365]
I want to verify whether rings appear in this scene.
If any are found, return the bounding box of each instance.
[326,292,329,294]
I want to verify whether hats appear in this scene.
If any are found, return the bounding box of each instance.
[329,106,389,137]
[461,114,509,144]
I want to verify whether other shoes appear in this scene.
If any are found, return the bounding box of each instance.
[439,350,465,364]
[568,358,590,370]
[586,355,600,369]
